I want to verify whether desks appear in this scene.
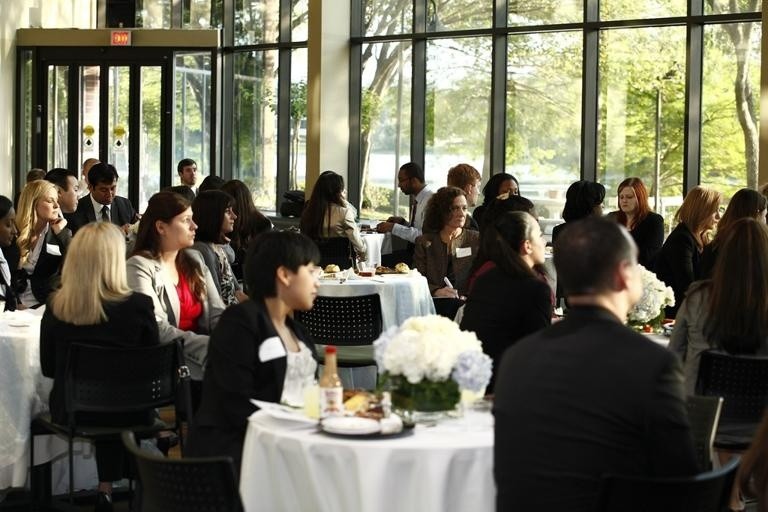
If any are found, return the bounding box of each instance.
[1,220,692,511]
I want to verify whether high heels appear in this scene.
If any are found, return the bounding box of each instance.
[156,436,178,458]
[96,492,113,510]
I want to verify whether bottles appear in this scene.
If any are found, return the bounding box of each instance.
[320,345,343,417]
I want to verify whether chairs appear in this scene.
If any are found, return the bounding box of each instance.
[687,394,744,463]
[694,351,768,452]
[598,455,746,512]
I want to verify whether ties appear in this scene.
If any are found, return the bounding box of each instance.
[100,206,110,223]
[410,200,416,227]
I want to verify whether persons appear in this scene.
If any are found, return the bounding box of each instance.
[656,186,767,510]
[38,221,161,511]
[492,215,692,510]
[0,159,275,397]
[295,170,368,271]
[376,162,555,401]
[552,177,665,275]
[203,230,323,477]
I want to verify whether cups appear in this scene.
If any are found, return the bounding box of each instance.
[302,379,344,420]
[369,218,378,230]
[355,258,377,276]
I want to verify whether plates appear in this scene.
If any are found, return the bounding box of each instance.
[8,320,31,326]
[318,417,379,434]
